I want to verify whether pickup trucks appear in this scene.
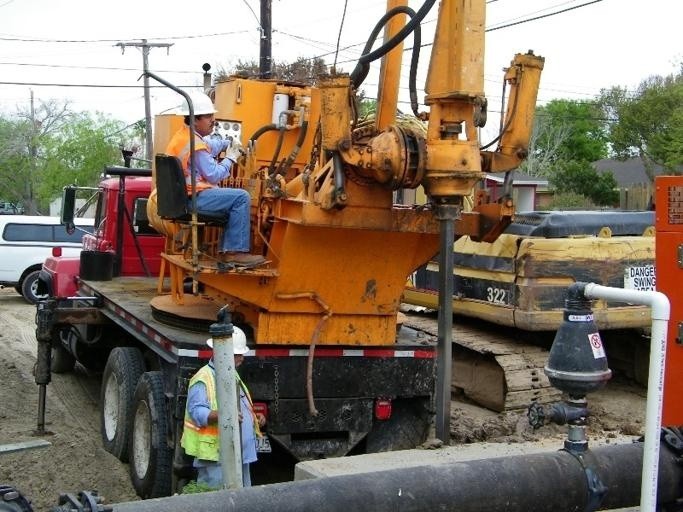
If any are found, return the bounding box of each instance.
[0,203,25,214]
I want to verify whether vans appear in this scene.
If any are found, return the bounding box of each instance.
[1,214,96,304]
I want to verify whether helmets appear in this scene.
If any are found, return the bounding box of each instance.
[207,323,251,355]
[174,91,217,117]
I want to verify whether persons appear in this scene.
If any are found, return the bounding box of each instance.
[162,89,267,270]
[177,325,267,493]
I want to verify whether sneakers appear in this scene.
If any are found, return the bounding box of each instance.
[217,246,267,268]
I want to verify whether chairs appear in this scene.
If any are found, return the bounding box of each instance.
[155,153,229,264]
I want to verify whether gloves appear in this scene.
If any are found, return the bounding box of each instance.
[224,135,246,164]
